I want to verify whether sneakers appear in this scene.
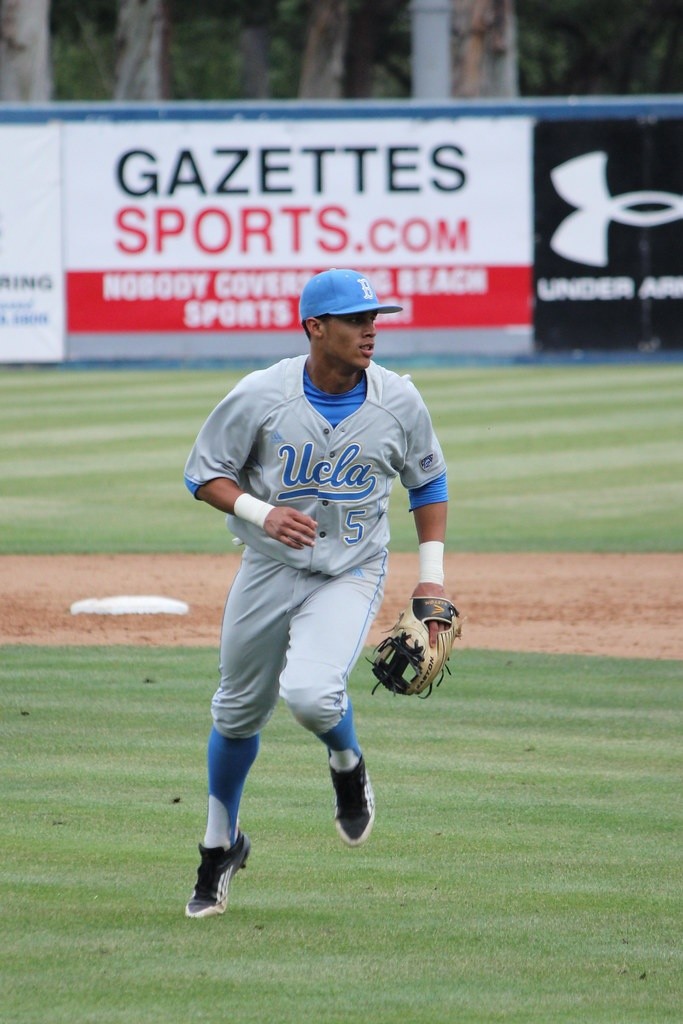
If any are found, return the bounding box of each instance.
[328,749,376,845]
[185,831,252,918]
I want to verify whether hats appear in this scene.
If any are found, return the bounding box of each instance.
[298,267,402,323]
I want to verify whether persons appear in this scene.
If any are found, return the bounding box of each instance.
[183,267,450,918]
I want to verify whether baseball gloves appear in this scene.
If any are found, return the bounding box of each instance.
[365,597,462,700]
